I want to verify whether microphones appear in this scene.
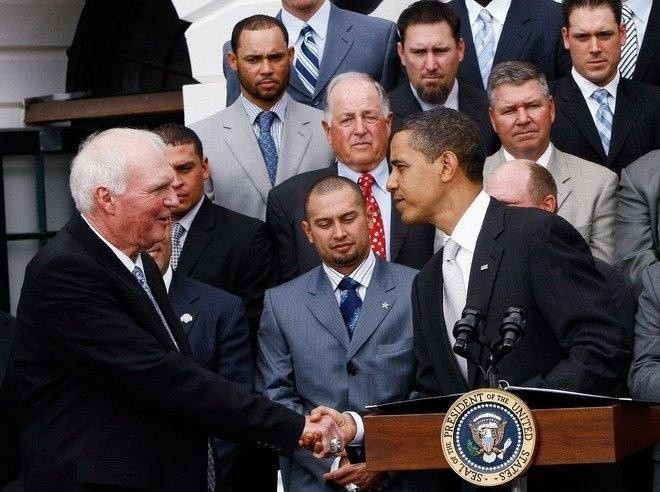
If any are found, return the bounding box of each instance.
[452,295,490,356]
[499,293,529,355]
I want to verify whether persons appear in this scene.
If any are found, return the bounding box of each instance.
[223,0,409,111]
[265,73,434,289]
[387,0,503,155]
[486,160,558,216]
[145,215,253,478]
[2,127,345,490]
[627,260,659,492]
[254,176,422,492]
[434,62,619,265]
[612,149,660,308]
[298,108,631,458]
[152,124,281,491]
[618,0,660,88]
[546,0,659,189]
[186,14,334,222]
[445,0,574,93]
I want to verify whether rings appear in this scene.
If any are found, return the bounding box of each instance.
[330,438,344,453]
[344,482,359,492]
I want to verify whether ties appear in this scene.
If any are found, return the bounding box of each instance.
[255,112,277,188]
[338,278,364,341]
[132,267,179,350]
[358,174,386,262]
[442,238,468,381]
[475,10,495,90]
[591,90,614,156]
[619,4,639,80]
[171,222,185,270]
[296,24,319,95]
